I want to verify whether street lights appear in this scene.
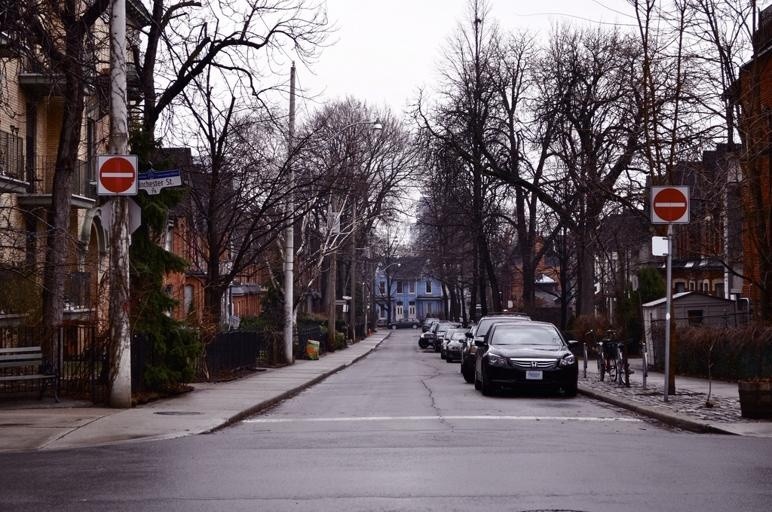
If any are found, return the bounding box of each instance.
[281,116,389,365]
[370,259,404,337]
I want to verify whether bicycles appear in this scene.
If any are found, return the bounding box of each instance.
[584,326,649,390]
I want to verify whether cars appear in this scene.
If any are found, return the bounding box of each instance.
[415,312,580,398]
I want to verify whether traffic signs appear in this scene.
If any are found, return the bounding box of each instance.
[136,174,190,197]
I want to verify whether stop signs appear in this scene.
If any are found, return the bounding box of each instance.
[99,158,136,192]
[653,187,688,221]
